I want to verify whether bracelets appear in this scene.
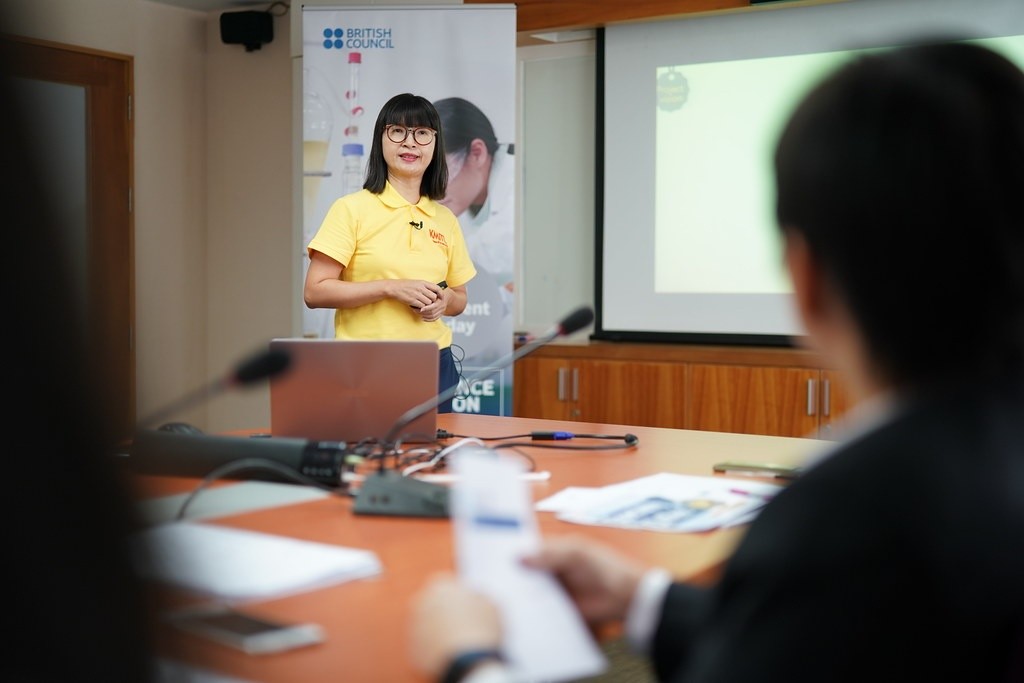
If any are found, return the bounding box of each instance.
[620,565,676,653]
[434,646,505,682]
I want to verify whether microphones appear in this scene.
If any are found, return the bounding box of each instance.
[353,307,595,518]
[112,348,294,452]
[410,221,423,230]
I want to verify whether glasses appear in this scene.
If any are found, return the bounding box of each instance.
[384,124,437,146]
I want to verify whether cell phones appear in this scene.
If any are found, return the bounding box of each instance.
[163,603,324,655]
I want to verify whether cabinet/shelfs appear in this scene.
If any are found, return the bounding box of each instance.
[508,343,877,440]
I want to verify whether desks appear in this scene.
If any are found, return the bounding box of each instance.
[109,410,840,683]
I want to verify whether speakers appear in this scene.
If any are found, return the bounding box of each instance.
[219,10,274,44]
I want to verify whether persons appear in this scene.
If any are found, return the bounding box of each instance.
[303,93,480,415]
[405,32,1024,680]
[426,97,515,276]
